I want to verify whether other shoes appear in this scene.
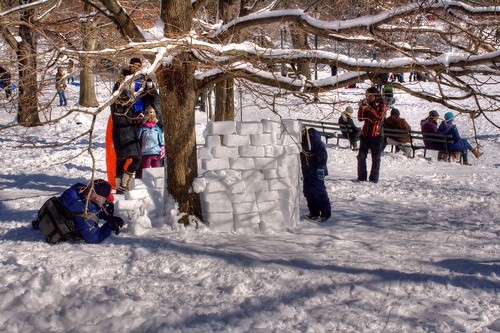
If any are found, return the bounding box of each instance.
[318,215,329,221]
[304,214,319,220]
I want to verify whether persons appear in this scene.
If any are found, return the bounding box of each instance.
[300,128,331,223]
[0,67,11,98]
[357,87,387,184]
[437,112,484,166]
[32,179,124,244]
[383,109,412,154]
[67,59,73,85]
[56,66,67,106]
[338,106,362,150]
[377,71,430,91]
[420,110,448,162]
[109,58,165,195]
[383,83,398,106]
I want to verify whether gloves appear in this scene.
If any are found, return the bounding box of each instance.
[316,168,325,180]
[108,216,125,228]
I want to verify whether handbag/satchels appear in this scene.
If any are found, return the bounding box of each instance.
[37,197,75,244]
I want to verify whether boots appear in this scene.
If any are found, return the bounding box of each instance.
[463,160,472,165]
[114,176,128,194]
[121,171,136,189]
[473,151,484,158]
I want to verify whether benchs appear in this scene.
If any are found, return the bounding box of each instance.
[297,118,352,149]
[383,128,466,163]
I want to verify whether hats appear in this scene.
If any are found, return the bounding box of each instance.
[445,112,453,121]
[144,107,156,117]
[346,106,353,113]
[129,58,142,67]
[94,179,112,197]
[391,108,400,115]
[429,110,439,116]
